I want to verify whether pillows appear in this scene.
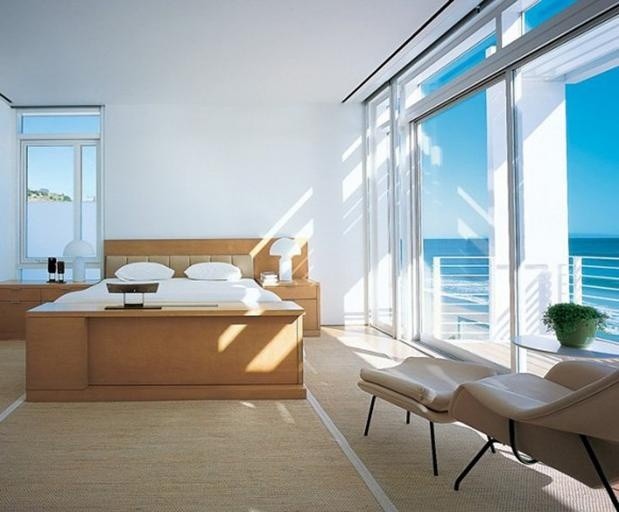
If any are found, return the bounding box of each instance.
[113,261,242,282]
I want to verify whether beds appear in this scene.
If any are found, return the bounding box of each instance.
[24,238,309,400]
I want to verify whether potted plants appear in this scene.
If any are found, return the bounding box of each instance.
[542,303,609,347]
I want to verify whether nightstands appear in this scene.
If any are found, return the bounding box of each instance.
[1,279,94,340]
[255,278,320,337]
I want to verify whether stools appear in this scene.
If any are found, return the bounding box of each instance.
[358,355,511,477]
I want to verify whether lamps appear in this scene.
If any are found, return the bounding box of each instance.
[269,238,302,281]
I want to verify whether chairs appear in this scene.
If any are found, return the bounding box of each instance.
[449,361,619,512]
